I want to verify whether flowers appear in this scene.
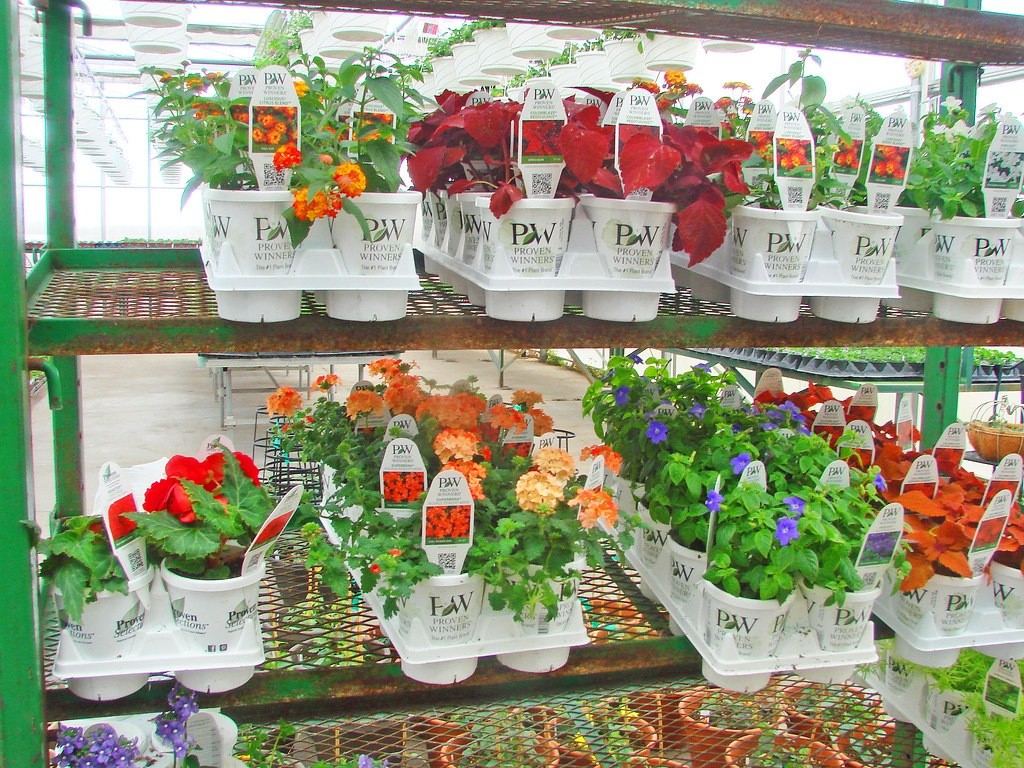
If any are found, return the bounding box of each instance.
[48,684,398,768]
[124,9,1023,271]
[18,359,1024,626]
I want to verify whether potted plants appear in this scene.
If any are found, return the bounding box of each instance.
[411,635,1024,768]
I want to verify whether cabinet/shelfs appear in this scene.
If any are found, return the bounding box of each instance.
[0,0,1024,768]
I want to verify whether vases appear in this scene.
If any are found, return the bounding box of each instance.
[55,708,250,768]
[197,180,1022,325]
[51,459,1024,695]
